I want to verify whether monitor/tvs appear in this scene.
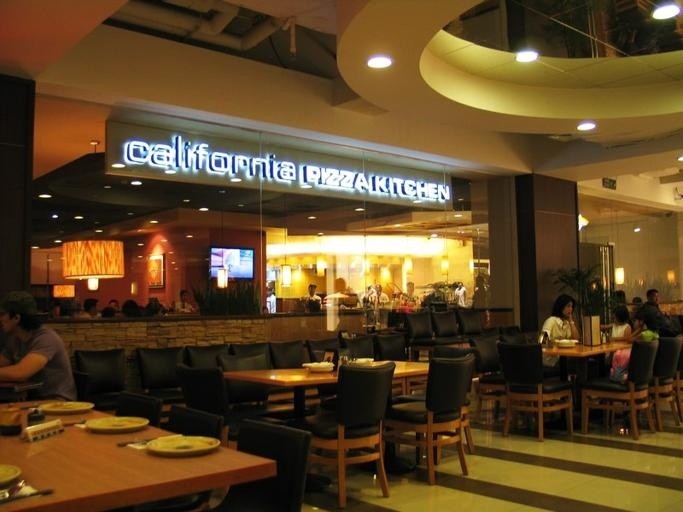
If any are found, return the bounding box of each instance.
[208,245,255,280]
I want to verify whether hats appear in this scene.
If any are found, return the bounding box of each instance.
[1,290,51,316]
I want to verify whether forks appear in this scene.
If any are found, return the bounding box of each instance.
[0,480,25,499]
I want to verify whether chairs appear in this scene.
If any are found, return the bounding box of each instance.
[500,331,529,349]
[382,353,476,486]
[75,349,127,416]
[129,405,229,512]
[302,362,396,509]
[392,346,481,465]
[136,346,187,423]
[186,332,404,415]
[174,363,283,450]
[404,308,521,361]
[202,419,312,511]
[72,369,90,403]
[216,354,295,415]
[611,335,683,431]
[116,391,164,427]
[498,343,574,442]
[470,333,507,424]
[581,338,659,441]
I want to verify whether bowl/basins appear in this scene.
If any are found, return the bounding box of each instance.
[356,358,374,364]
[555,340,576,347]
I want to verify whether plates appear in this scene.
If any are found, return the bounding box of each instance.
[146,435,221,458]
[86,416,150,433]
[310,367,333,371]
[38,401,95,412]
[0,464,22,485]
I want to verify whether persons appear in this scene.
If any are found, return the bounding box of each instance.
[176,289,196,312]
[601,304,631,383]
[368,284,388,303]
[0,291,77,403]
[641,289,680,338]
[101,306,116,318]
[540,294,578,379]
[302,284,323,311]
[266,287,276,313]
[77,299,100,317]
[629,310,658,344]
[456,282,467,306]
[323,278,351,311]
[400,281,419,305]
[109,299,119,312]
[121,300,139,318]
[471,276,487,310]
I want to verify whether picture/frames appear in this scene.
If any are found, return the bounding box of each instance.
[147,253,166,289]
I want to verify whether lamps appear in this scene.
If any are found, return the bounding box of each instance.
[62,240,125,279]
[88,279,99,291]
[615,209,625,285]
[53,285,75,297]
[281,198,292,287]
[217,195,228,288]
